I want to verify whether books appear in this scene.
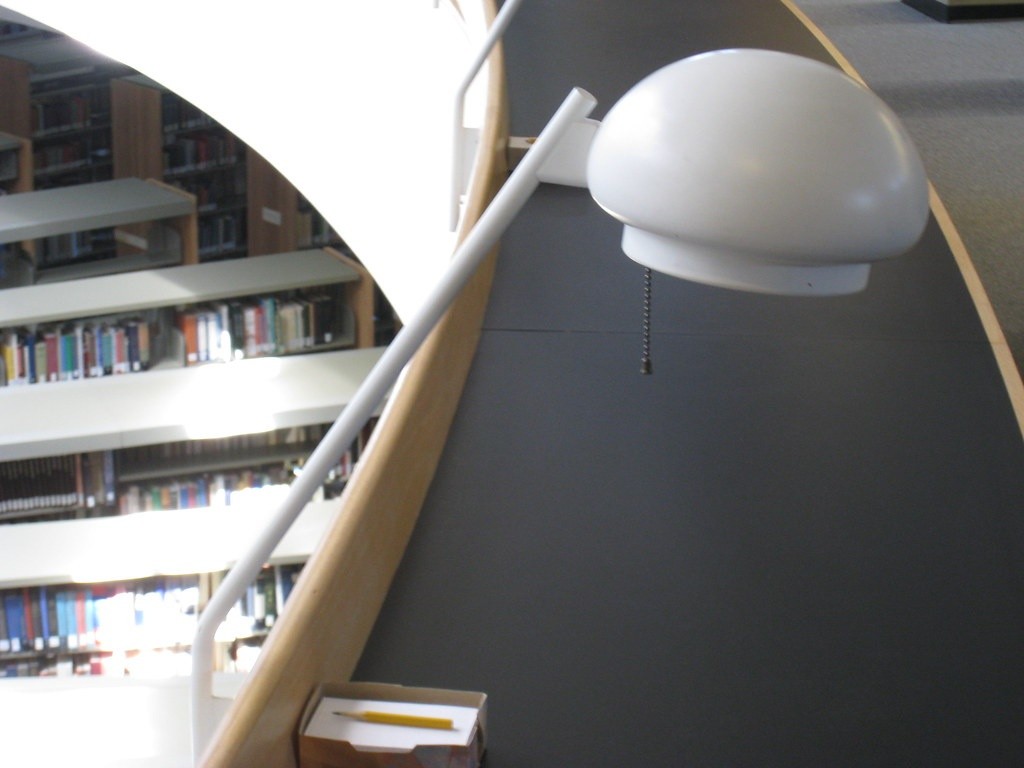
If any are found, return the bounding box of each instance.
[0,418,376,525]
[0,292,336,387]
[0,72,341,270]
[0,564,305,677]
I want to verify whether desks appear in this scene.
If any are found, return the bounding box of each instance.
[200,0,1024,768]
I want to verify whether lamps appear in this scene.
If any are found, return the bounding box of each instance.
[189,48,934,768]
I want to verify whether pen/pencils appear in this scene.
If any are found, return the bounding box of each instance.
[331,710,453,730]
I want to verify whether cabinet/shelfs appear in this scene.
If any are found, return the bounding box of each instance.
[0,57,404,674]
